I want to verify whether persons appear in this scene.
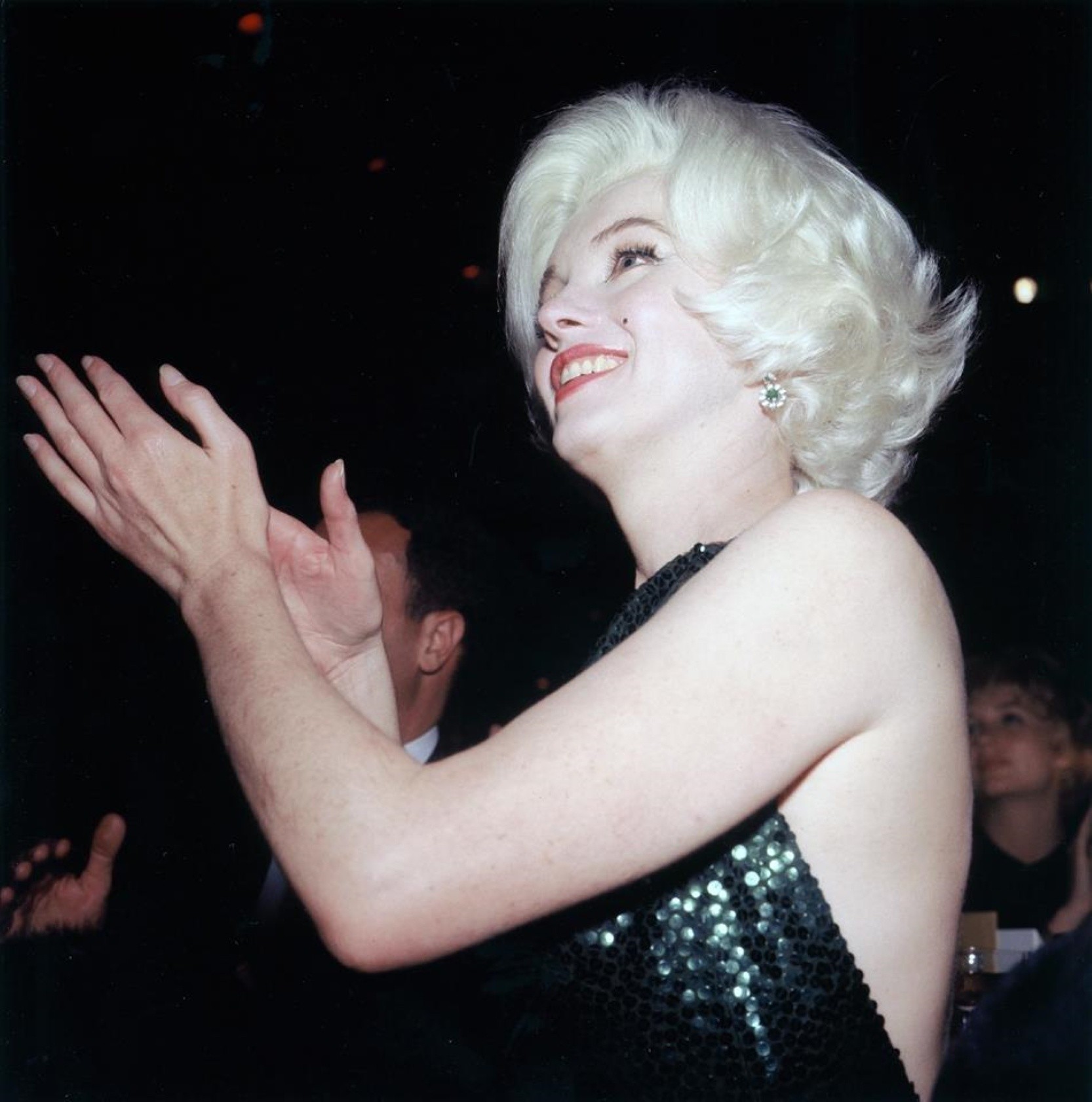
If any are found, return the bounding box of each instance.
[8,82,981,1102]
[955,654,1092,1101]
[0,470,503,1101]
